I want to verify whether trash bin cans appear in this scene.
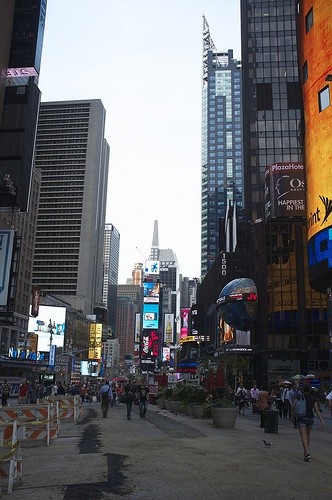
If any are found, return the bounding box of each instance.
[264,410,279,434]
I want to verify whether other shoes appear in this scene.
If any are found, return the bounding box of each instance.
[304,454,311,462]
[304,453,306,457]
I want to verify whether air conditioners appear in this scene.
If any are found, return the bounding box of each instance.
[318,360,330,369]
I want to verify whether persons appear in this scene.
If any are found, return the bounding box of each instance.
[0,380,332,419]
[100,381,113,417]
[291,384,325,460]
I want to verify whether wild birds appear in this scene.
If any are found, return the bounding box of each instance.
[262,439,272,448]
[155,410,178,418]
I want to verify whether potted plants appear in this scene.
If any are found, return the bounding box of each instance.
[157,344,250,430]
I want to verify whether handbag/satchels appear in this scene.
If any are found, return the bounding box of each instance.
[102,392,108,398]
[292,393,306,418]
